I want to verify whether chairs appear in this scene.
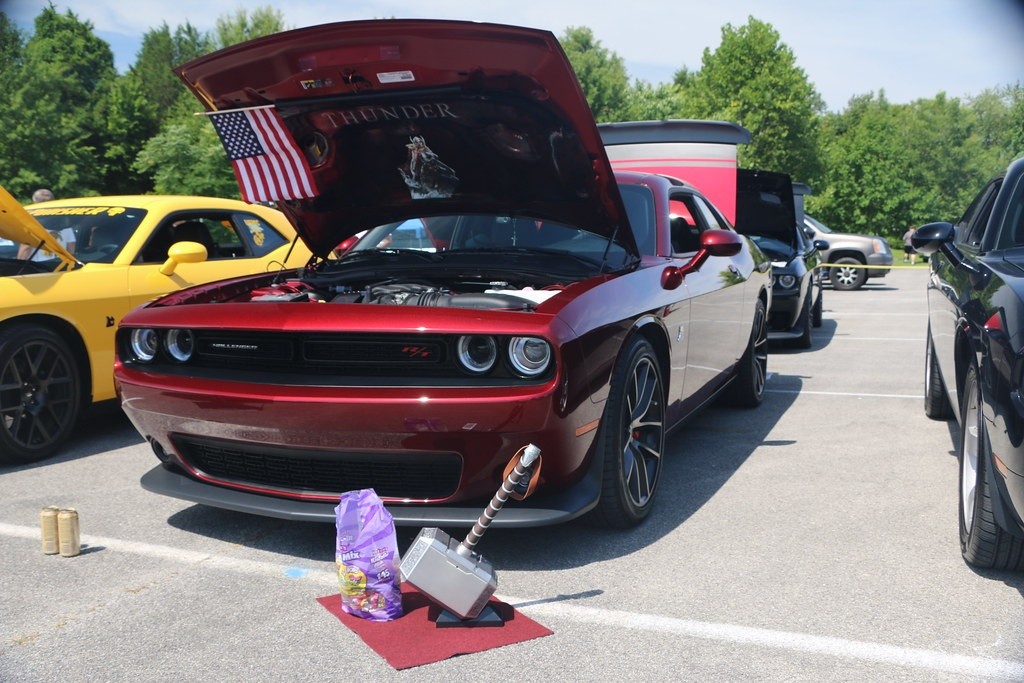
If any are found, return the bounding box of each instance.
[669,213,693,253]
[173,220,215,259]
[489,218,580,247]
[76,228,116,261]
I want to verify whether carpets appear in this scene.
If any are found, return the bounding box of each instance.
[316,580,555,671]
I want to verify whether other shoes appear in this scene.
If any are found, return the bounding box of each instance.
[911,261,915,265]
[904,259,908,263]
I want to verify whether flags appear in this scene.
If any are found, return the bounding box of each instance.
[209,104,319,205]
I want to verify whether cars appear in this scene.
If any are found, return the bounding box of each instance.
[910,155,1024,572]
[803,213,892,292]
[734,169,824,351]
[0,184,339,465]
[110,15,774,533]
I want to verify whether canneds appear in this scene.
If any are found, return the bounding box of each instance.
[40,505,60,555]
[57,508,80,556]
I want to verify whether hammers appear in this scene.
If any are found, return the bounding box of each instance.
[398,443,541,628]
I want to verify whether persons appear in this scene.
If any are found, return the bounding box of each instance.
[903,226,917,265]
[18,188,76,262]
